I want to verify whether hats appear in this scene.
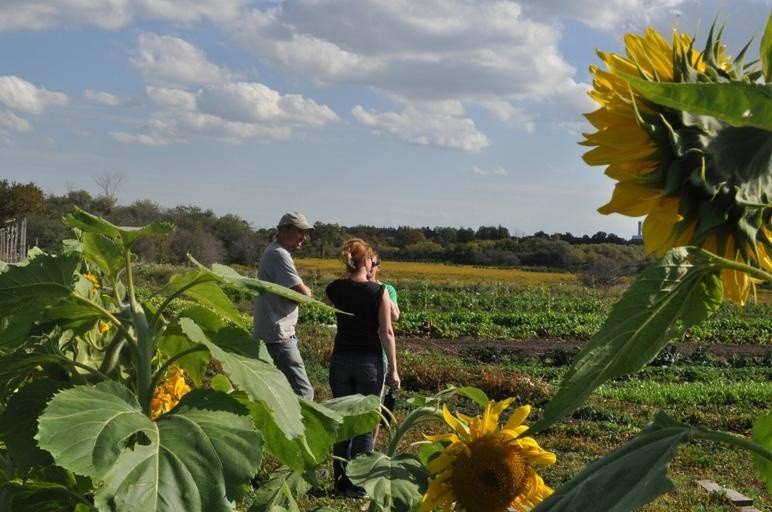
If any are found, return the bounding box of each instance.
[277,210,315,230]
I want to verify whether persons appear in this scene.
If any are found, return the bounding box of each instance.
[253,209,317,403]
[322,237,402,500]
[366,249,400,426]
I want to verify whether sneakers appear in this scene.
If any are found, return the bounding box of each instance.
[334,486,369,498]
